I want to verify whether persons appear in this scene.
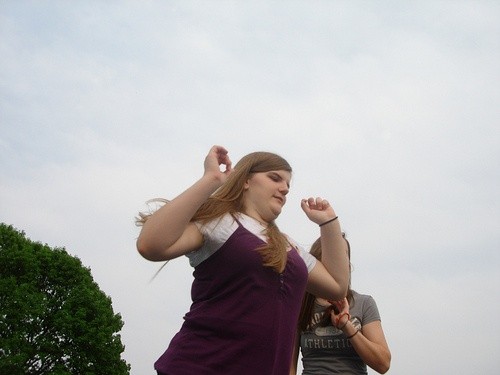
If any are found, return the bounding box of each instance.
[136,145,349,375]
[289,232,391,375]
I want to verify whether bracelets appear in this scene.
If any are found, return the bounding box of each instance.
[347,330,360,340]
[319,216,338,227]
[333,311,350,329]
[340,320,348,330]
